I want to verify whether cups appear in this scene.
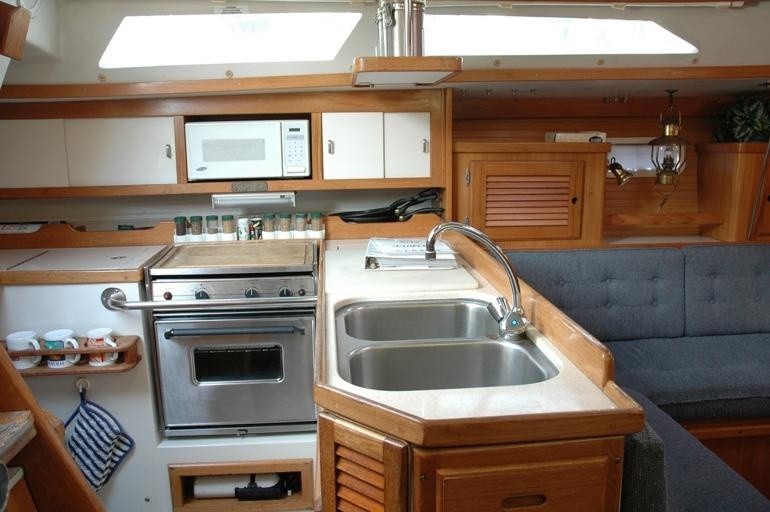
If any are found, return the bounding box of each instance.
[44,329,81,369]
[5,331,42,370]
[85,328,119,366]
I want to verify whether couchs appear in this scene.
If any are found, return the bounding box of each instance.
[496,244,770,512]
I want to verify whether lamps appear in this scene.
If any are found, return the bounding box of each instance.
[648,89,692,215]
[607,155,633,186]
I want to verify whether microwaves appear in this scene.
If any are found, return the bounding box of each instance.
[184,119,311,181]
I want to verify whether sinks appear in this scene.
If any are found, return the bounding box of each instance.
[337,342,558,392]
[334,296,528,344]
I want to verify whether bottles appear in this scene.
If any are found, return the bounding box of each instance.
[174,211,323,241]
[392,0,408,57]
[377,0,393,57]
[408,0,425,57]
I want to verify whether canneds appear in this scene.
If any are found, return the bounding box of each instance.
[311,211,322,230]
[296,212,306,230]
[190,216,203,235]
[250,218,262,240]
[223,216,235,233]
[206,215,217,234]
[279,213,291,231]
[237,218,250,240]
[174,216,186,235]
[263,213,275,232]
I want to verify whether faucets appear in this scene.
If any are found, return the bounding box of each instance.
[425,223,528,341]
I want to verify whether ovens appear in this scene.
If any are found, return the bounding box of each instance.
[143,277,321,438]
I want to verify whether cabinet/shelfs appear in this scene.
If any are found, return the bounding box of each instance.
[454,141,611,250]
[436,456,607,512]
[321,110,384,179]
[0,118,70,188]
[64,116,178,186]
[317,412,408,512]
[384,112,430,179]
